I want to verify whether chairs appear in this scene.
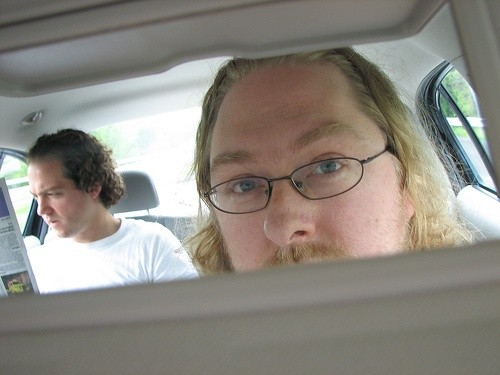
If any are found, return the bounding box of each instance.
[107,168,174,237]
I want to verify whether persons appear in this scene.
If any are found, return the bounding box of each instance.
[177,47,486,278]
[22,127,199,294]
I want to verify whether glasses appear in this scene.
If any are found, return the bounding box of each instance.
[203,144,390,216]
